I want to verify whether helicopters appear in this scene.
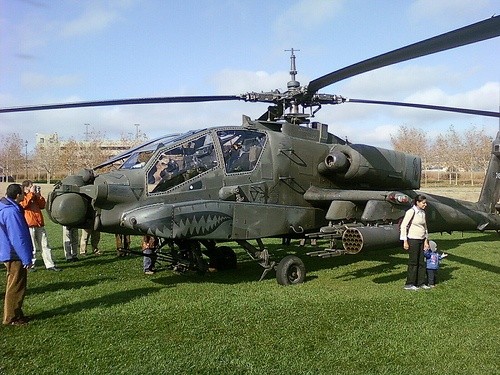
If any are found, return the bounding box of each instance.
[0,15,500,286]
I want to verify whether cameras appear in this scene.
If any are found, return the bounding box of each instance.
[35,187,40,194]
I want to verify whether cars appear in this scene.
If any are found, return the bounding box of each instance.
[422,164,460,179]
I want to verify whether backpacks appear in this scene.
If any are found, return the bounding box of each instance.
[397,208,415,240]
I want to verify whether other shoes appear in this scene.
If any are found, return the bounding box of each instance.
[3,316,33,326]
[67,257,80,262]
[417,283,430,289]
[404,284,419,290]
[49,266,62,271]
[80,253,86,257]
[145,271,154,275]
[30,268,36,272]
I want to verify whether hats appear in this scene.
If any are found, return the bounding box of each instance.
[429,240,437,249]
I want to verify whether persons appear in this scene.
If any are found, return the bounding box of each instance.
[80,228,104,256]
[399,194,429,290]
[424,241,447,287]
[18,180,63,273]
[62,224,81,263]
[115,234,131,258]
[142,236,159,274]
[0,183,34,327]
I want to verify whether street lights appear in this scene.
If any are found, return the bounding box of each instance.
[24,139,29,178]
[83,123,91,140]
[134,123,139,142]
